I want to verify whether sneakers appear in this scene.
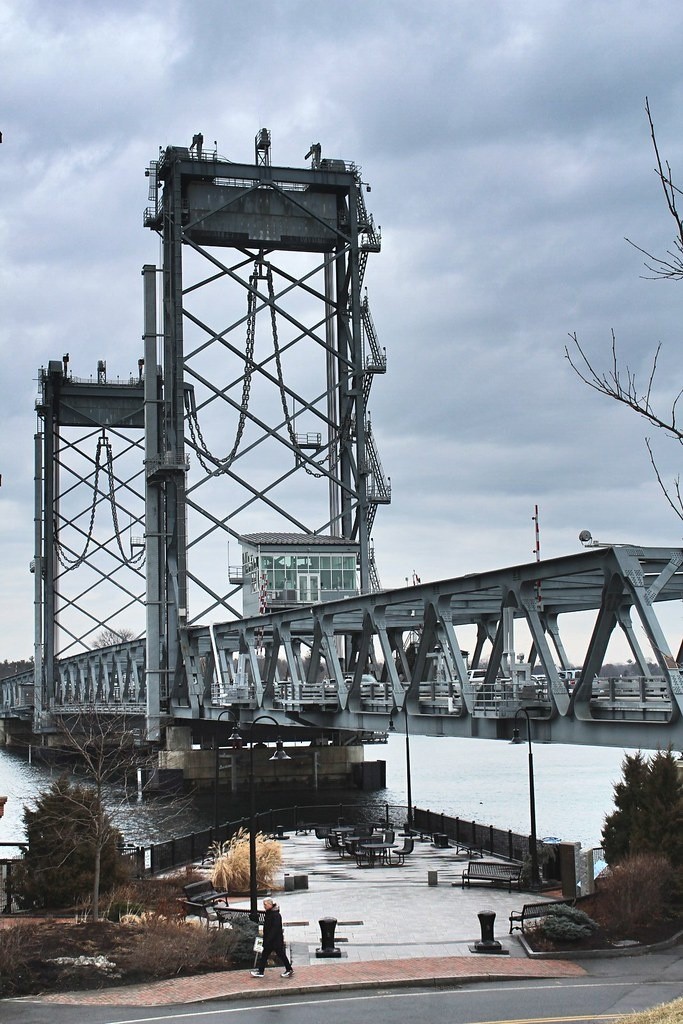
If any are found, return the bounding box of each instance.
[280,969,294,978]
[250,970,265,977]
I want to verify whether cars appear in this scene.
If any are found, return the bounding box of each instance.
[319,679,337,692]
[529,675,548,701]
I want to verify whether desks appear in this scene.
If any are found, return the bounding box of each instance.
[331,828,354,845]
[361,843,399,868]
[344,836,382,861]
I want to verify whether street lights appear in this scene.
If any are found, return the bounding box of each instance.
[387,705,414,832]
[216,709,244,850]
[250,716,292,933]
[509,708,543,892]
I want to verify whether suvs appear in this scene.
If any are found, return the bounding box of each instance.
[558,669,600,698]
[344,674,384,695]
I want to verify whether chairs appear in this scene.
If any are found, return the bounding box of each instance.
[313,823,414,868]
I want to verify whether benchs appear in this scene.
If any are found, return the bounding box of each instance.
[295,823,317,835]
[454,843,483,859]
[177,898,219,930]
[462,861,523,894]
[214,908,266,930]
[509,892,600,935]
[183,879,229,908]
[409,827,433,844]
[202,850,228,866]
[372,823,394,832]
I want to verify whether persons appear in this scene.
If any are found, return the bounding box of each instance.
[251,897,295,978]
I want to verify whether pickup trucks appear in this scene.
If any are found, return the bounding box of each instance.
[467,669,498,690]
